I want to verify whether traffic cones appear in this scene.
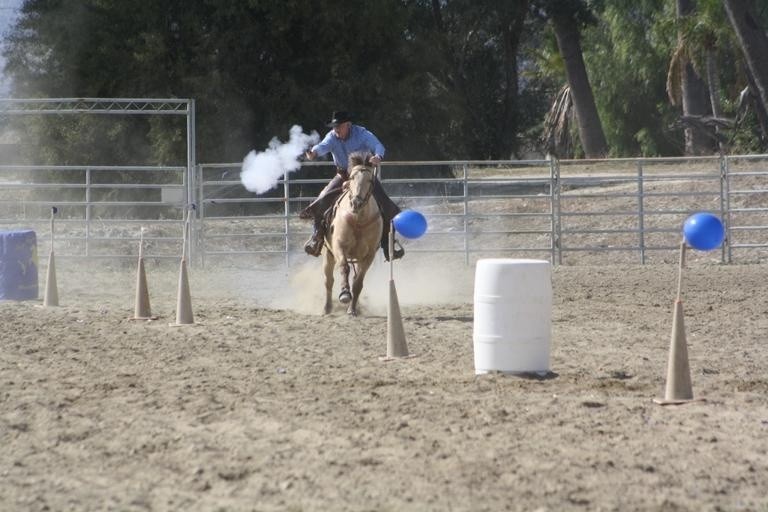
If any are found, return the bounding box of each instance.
[176,260,194,324]
[385,281,410,357]
[135,258,152,317]
[43,250,59,306]
[665,302,694,400]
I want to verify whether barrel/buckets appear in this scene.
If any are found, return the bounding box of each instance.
[472,258,553,379]
[0,227,38,300]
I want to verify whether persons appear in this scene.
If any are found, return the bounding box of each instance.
[302,110,404,259]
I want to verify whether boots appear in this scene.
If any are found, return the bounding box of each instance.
[305,216,324,257]
[383,228,404,262]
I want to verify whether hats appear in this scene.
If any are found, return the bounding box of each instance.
[327,110,353,127]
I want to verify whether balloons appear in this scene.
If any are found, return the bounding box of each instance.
[392,208,427,240]
[682,212,724,252]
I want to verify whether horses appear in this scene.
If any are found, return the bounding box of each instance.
[317,150,386,314]
